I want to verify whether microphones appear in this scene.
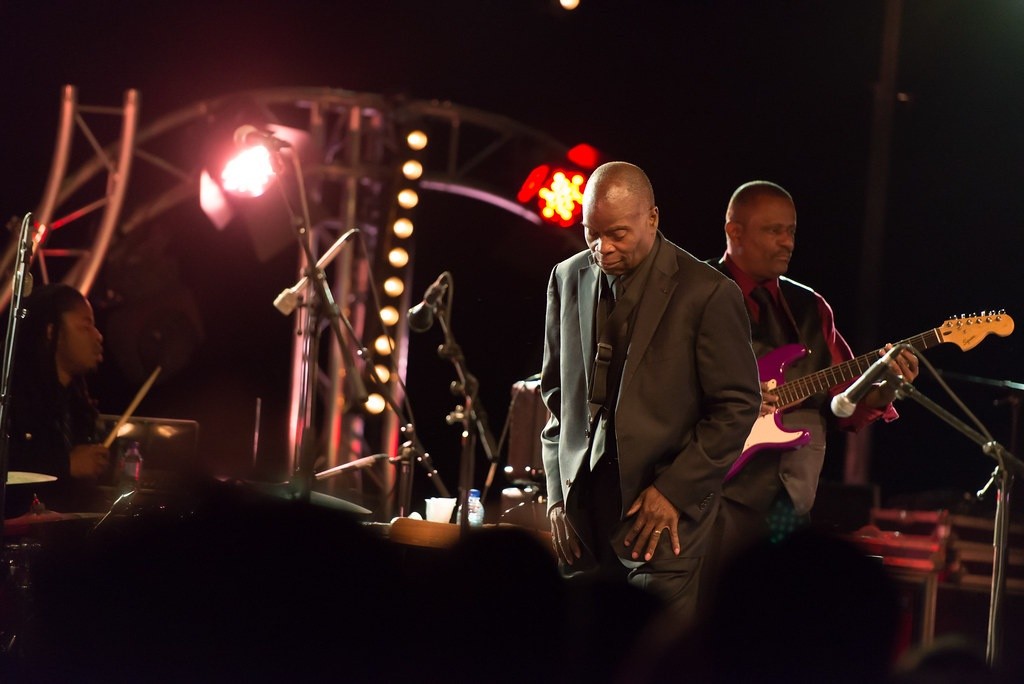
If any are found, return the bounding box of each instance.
[315,454,387,481]
[274,230,358,316]
[408,273,448,332]
[831,345,903,417]
[12,215,34,298]
[232,125,291,150]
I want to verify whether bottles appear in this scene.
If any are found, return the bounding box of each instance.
[457,489,484,528]
[930,505,958,584]
[121,440,144,512]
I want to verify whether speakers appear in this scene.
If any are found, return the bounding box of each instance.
[509,382,551,481]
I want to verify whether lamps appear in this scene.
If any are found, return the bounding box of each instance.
[205,124,278,201]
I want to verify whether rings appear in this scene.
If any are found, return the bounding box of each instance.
[652,529,661,534]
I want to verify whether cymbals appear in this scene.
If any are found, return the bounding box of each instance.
[219,470,376,518]
[2,466,59,486]
[2,494,112,533]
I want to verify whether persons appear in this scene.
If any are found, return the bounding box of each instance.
[0,283,125,518]
[704,180,919,683]
[539,162,761,684]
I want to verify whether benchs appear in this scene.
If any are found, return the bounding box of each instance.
[864,506,1024,650]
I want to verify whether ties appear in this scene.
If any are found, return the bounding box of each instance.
[56,385,72,453]
[590,274,626,474]
[748,284,788,349]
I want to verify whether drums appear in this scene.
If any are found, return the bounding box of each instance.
[3,541,47,616]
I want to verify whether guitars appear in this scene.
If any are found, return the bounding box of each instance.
[724,304,1017,485]
